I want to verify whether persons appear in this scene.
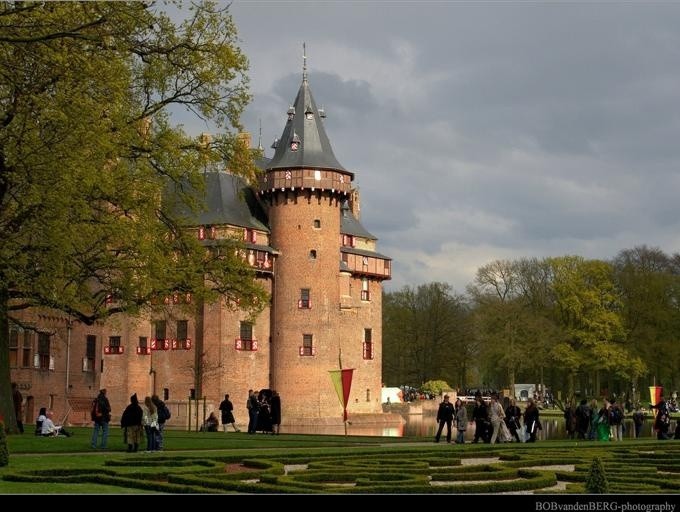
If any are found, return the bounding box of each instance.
[41,411,75,438]
[88,388,112,450]
[218,393,241,433]
[246,388,283,434]
[11,381,25,434]
[141,395,161,453]
[198,411,220,431]
[151,394,166,451]
[394,383,680,444]
[119,391,144,453]
[34,407,64,434]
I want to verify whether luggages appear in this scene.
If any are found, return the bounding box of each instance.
[511,417,526,443]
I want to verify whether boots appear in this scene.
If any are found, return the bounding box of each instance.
[133,443,138,452]
[126,444,132,452]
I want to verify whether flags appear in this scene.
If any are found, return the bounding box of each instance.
[327,368,356,422]
